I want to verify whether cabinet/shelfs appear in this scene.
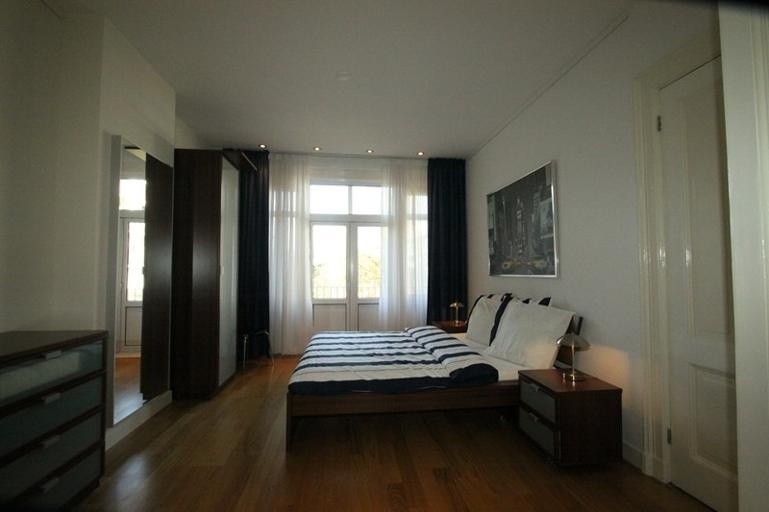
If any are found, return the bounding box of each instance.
[0,330,112,510]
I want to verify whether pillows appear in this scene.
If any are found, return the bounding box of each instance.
[464,291,577,378]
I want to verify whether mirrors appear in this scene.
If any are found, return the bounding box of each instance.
[107,130,174,427]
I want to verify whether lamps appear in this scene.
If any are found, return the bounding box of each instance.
[449,301,464,324]
[555,331,590,381]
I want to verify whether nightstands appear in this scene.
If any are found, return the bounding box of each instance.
[516,369,624,471]
[439,320,465,331]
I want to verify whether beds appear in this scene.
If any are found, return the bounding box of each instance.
[283,302,584,425]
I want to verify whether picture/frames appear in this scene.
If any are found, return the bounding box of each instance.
[484,159,559,279]
